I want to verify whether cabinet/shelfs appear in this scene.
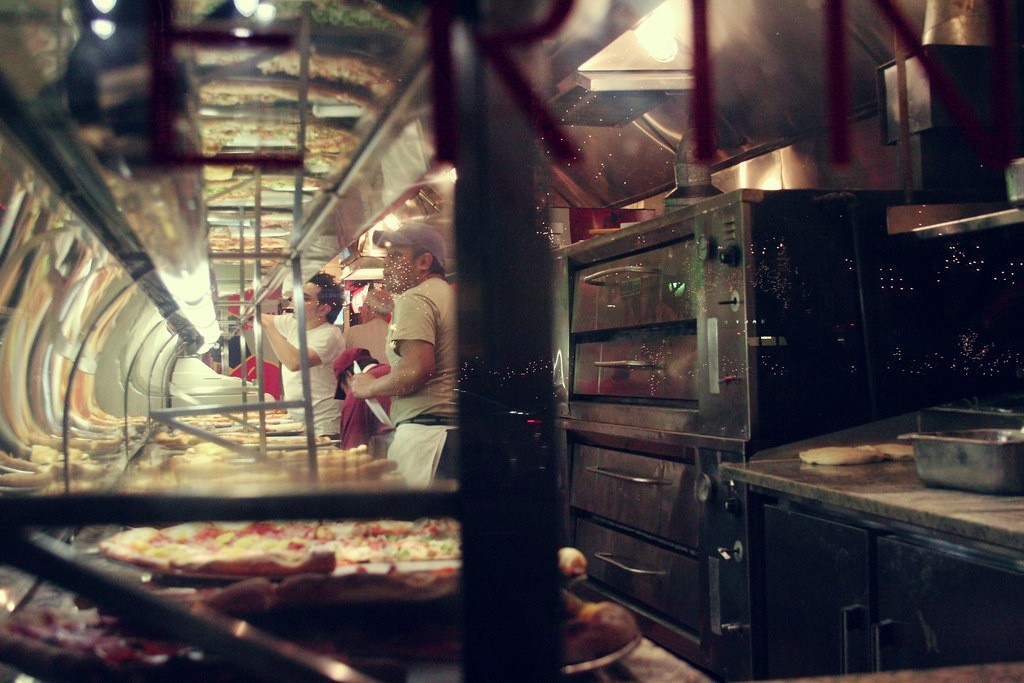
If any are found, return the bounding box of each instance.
[763,504,1024,679]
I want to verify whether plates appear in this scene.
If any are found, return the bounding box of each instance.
[353,360,392,425]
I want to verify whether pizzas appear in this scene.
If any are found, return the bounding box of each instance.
[132,410,398,488]
[96,518,465,579]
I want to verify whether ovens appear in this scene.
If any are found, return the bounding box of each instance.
[551,189,922,683]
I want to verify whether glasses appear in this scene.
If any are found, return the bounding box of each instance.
[359,307,366,312]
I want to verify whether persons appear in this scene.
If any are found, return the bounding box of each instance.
[209,223,460,481]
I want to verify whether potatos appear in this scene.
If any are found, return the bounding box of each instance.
[562,590,635,666]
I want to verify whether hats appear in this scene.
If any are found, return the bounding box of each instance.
[333,348,372,399]
[373,224,447,273]
